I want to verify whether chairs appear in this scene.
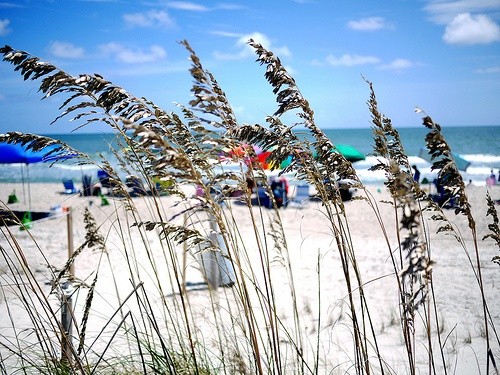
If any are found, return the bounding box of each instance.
[63,179,76,194]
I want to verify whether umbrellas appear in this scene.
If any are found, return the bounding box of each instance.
[419,147,470,172]
[246,142,296,169]
[385,156,430,169]
[311,144,365,162]
[205,141,254,160]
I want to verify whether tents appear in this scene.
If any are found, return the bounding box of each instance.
[0,136,86,222]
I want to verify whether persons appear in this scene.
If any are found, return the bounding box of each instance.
[411,165,420,195]
[490,169,500,186]
